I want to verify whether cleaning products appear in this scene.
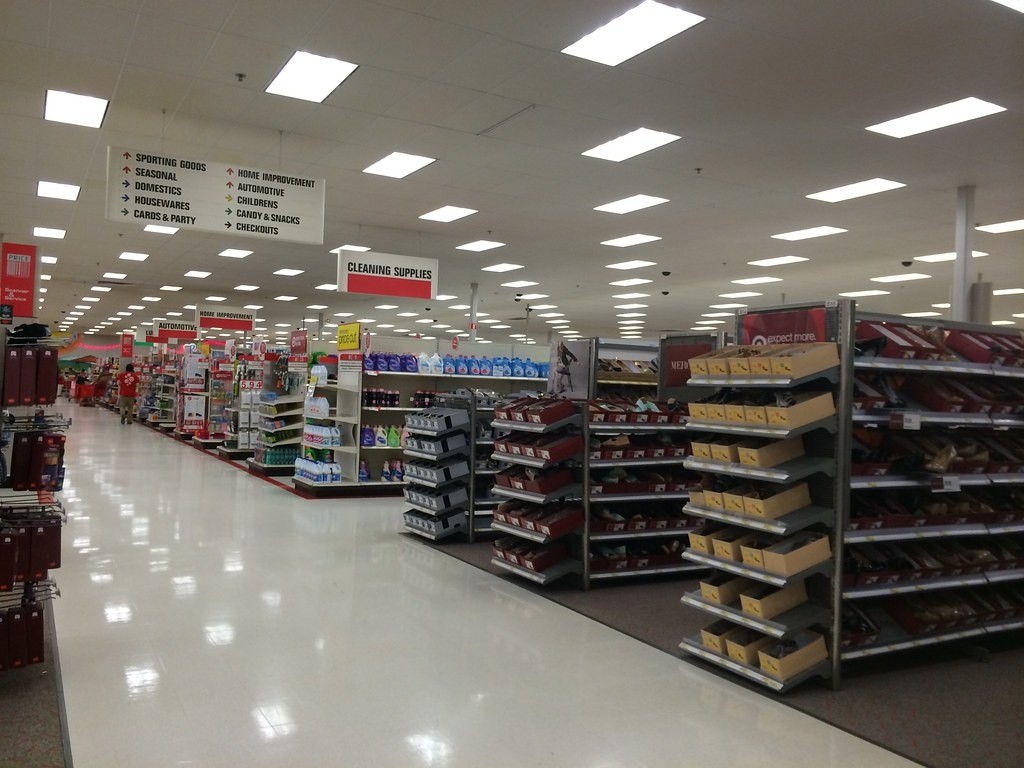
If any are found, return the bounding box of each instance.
[311,365,328,385]
[303,422,341,447]
[294,446,342,484]
[362,348,551,378]
[358,457,405,483]
[304,394,330,418]
[360,424,412,447]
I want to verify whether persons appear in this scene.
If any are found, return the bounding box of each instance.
[57,370,66,397]
[80,369,85,377]
[117,364,140,425]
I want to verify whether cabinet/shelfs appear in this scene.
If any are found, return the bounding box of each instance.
[292,354,548,496]
[679,301,1024,694]
[87,362,309,476]
[490,332,734,593]
[403,395,505,545]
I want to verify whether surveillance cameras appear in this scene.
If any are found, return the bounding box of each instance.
[426,307,431,310]
[54,321,58,323]
[365,328,368,330]
[662,291,670,295]
[902,261,912,267]
[341,321,344,323]
[662,272,671,276]
[61,311,66,313]
[514,293,534,314]
[435,320,437,322]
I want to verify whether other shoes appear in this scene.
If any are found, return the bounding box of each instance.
[121,415,125,424]
[128,421,132,424]
[495,320,1024,686]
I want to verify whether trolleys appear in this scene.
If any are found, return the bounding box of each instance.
[75,384,95,406]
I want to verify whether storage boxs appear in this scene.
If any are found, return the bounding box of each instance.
[688,319,1024,679]
[403,389,503,535]
[494,359,698,572]
